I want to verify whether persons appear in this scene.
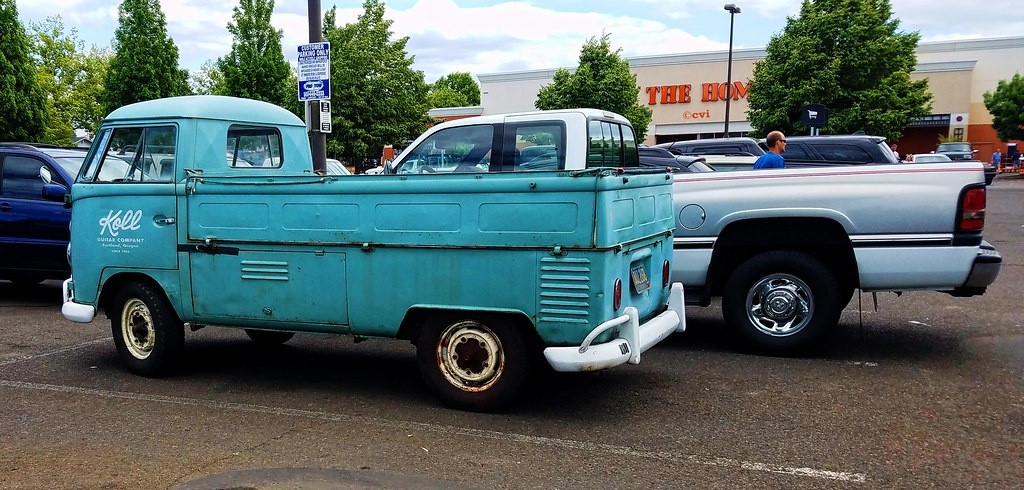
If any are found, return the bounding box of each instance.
[992,148,1002,174]
[890,142,913,164]
[753,131,786,170]
[1011,150,1024,171]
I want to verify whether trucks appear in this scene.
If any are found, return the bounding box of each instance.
[58,93,690,404]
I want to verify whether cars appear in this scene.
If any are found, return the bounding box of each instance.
[907,152,952,163]
[262,156,353,176]
[521,145,555,162]
[935,141,978,161]
[649,128,901,169]
[984,163,997,184]
[517,149,719,174]
[106,148,252,178]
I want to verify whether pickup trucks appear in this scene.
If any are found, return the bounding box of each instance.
[379,106,1003,354]
[364,158,484,173]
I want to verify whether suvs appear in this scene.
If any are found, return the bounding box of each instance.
[0,142,153,285]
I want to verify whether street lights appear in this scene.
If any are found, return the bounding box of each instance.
[724,3,741,138]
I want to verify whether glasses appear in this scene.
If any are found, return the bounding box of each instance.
[778,139,786,143]
